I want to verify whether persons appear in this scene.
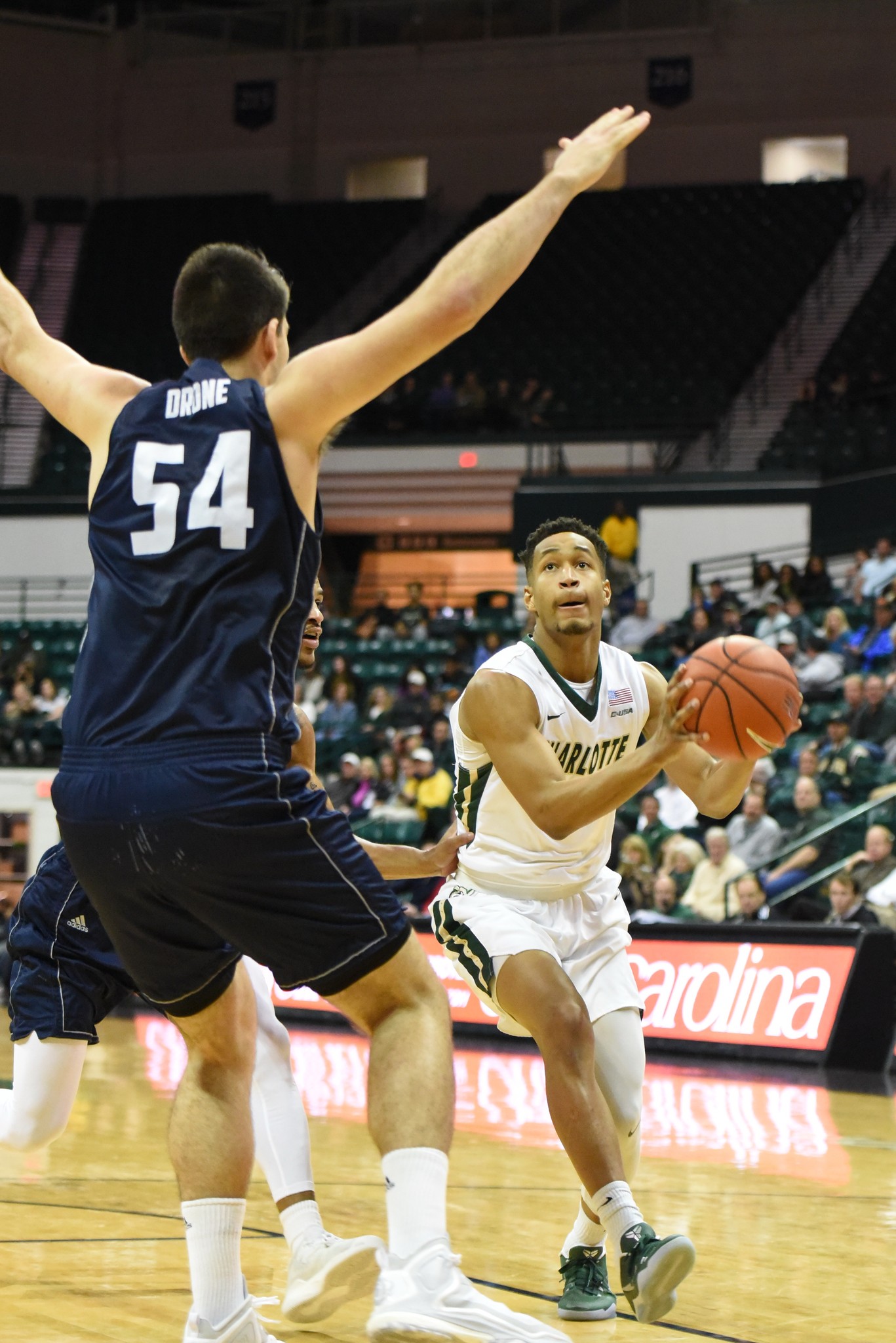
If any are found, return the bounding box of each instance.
[0,579,474,1322]
[0,538,896,997]
[598,501,640,561]
[433,515,803,1325]
[0,106,650,1343]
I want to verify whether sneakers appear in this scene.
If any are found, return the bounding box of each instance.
[559,1249,619,1322]
[283,1233,386,1324]
[182,1277,286,1342]
[621,1222,695,1323]
[366,1241,570,1343]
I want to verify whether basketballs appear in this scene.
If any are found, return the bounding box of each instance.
[679,632,805,763]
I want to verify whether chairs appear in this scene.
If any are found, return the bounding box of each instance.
[0,619,896,917]
